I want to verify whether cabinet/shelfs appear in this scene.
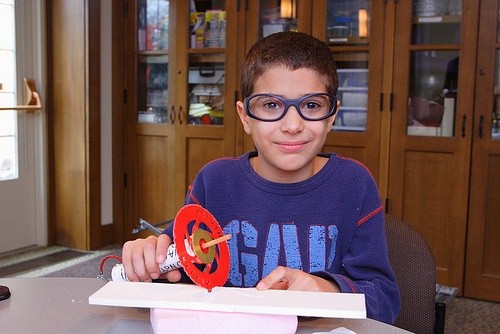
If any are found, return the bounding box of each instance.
[114,0,500,301]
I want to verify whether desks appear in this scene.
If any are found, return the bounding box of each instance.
[0,278,418,334]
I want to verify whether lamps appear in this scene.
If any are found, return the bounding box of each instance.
[280,0,296,19]
[359,9,368,37]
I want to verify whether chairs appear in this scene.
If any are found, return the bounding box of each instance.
[381,213,446,334]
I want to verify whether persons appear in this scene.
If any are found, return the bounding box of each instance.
[122,30,403,324]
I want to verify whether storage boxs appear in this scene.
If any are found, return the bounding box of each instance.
[332,69,369,131]
[189,70,226,83]
[205,9,226,25]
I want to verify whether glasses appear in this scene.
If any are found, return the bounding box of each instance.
[243,91,340,122]
